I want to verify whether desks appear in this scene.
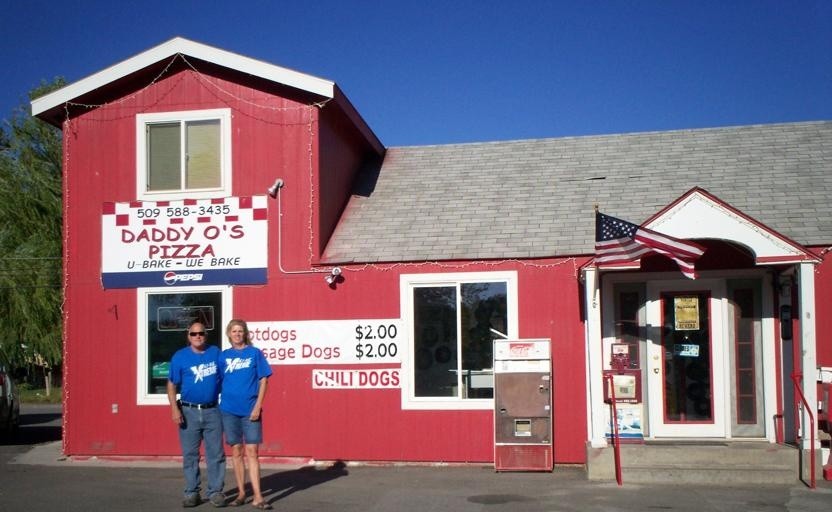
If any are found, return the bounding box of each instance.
[456,372,494,399]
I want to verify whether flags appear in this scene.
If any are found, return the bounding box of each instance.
[595,209,706,284]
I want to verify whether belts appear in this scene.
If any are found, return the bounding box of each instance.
[180,400,219,410]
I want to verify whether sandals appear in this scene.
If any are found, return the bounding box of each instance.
[252,500,271,510]
[228,498,243,506]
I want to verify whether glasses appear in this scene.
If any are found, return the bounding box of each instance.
[190,332,204,336]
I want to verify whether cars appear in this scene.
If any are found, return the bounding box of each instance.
[1,370,20,421]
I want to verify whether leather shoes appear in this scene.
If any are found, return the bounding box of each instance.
[210,494,227,507]
[183,495,201,507]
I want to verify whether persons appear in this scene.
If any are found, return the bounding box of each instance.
[218,319,274,510]
[167,321,228,508]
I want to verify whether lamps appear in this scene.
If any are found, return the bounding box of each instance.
[267,178,284,197]
[324,267,342,287]
